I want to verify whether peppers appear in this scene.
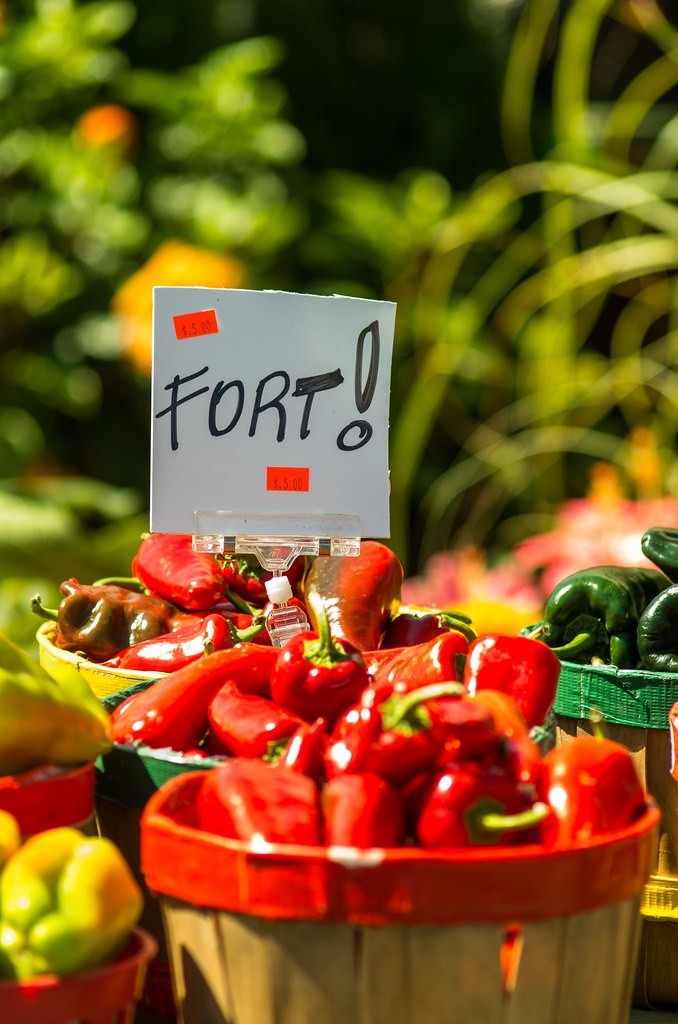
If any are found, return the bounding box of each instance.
[0,528,678,983]
